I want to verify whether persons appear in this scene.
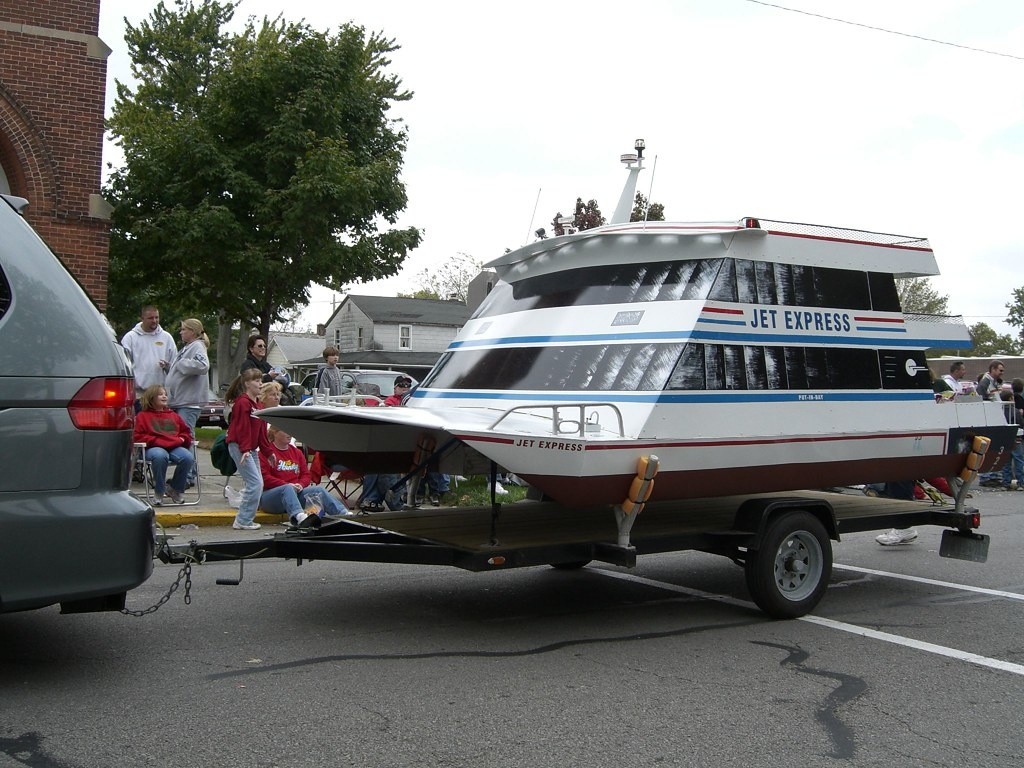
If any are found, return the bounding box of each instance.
[242,335,279,383]
[315,346,342,403]
[132,385,194,505]
[258,425,353,528]
[876,479,918,546]
[158,318,209,486]
[486,472,508,495]
[257,382,296,450]
[928,359,1024,491]
[119,305,178,417]
[311,375,450,513]
[223,368,277,530]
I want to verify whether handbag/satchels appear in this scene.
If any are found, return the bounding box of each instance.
[210,434,237,477]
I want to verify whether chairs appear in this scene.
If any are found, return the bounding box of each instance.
[308,448,364,510]
[128,399,202,508]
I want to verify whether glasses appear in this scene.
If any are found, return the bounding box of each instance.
[995,367,1003,372]
[395,383,412,388]
[254,345,267,348]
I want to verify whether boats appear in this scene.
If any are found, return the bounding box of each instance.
[253,137,1021,516]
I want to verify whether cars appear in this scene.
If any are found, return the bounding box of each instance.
[299,371,421,400]
[196,388,228,430]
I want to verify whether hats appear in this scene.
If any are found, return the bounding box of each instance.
[394,376,412,387]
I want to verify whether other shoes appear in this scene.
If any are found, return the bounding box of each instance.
[430,496,440,506]
[233,518,262,530]
[1017,485,1024,491]
[415,497,426,506]
[486,482,509,494]
[862,487,878,498]
[1001,485,1014,491]
[403,505,422,512]
[292,512,307,524]
[358,498,385,512]
[979,479,1002,487]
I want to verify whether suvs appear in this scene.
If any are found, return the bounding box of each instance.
[1,193,159,607]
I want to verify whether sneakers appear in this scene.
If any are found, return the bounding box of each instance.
[875,526,918,545]
[165,485,185,504]
[152,496,163,505]
